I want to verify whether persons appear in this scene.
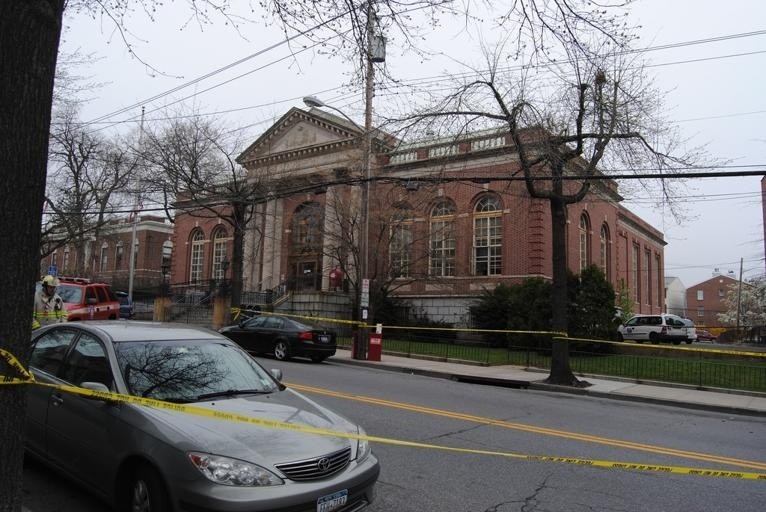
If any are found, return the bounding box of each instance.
[32,274,68,330]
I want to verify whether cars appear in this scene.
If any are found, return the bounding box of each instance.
[213,313,338,363]
[25,322,380,512]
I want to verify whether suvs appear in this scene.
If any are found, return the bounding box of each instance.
[684,319,699,344]
[619,314,687,344]
[39,277,121,323]
[109,290,133,317]
[696,330,717,343]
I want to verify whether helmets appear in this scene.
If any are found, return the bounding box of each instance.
[42,274,60,287]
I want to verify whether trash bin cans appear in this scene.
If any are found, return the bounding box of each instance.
[351,332,382,361]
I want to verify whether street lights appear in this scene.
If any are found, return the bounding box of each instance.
[300,97,371,366]
